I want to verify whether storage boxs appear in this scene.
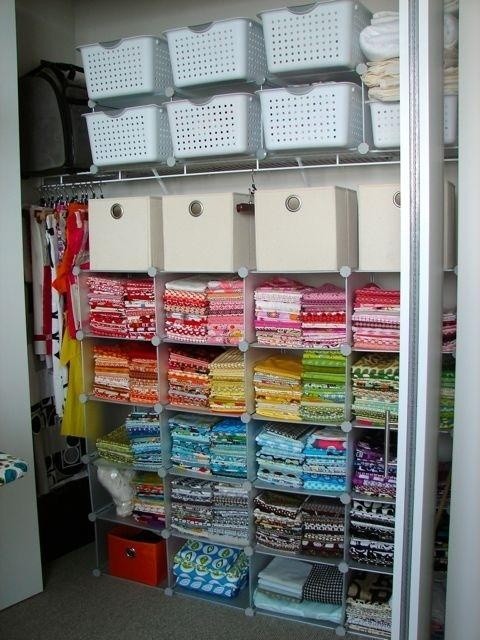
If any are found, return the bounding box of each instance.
[85,194,164,272]
[161,192,257,272]
[359,179,457,270]
[105,527,167,587]
[253,185,359,268]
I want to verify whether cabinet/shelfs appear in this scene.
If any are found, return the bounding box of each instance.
[73,267,454,638]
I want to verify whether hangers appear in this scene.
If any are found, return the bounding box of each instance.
[20,181,107,214]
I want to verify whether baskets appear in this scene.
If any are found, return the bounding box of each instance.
[78,0,456,167]
[367,88,456,145]
[81,102,172,169]
[258,1,373,84]
[253,82,372,154]
[158,16,288,98]
[76,34,172,107]
[161,92,262,160]
